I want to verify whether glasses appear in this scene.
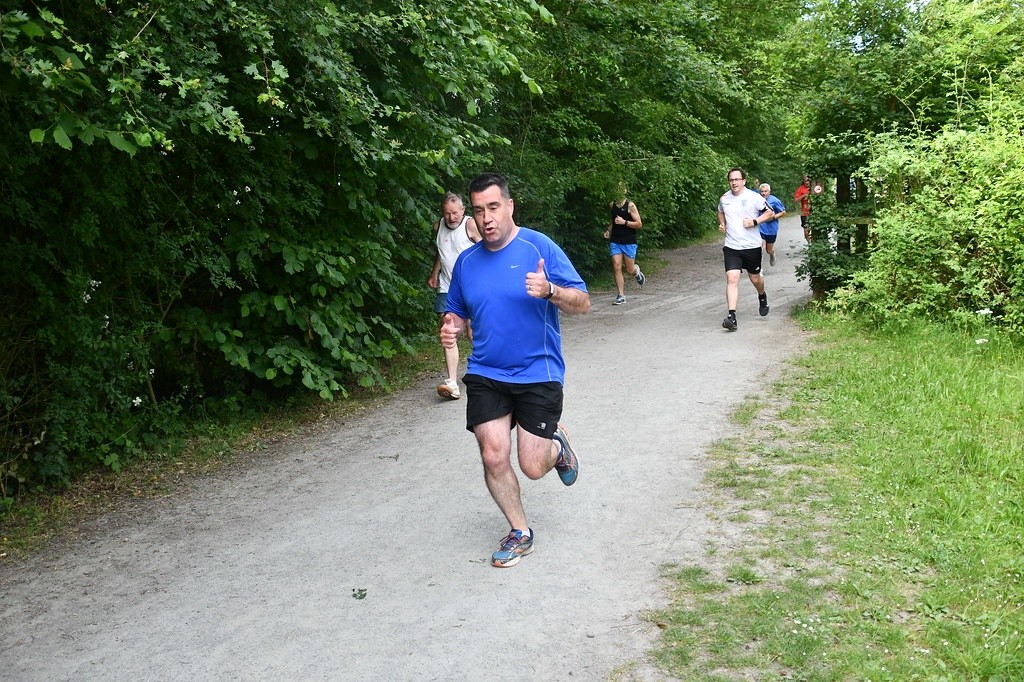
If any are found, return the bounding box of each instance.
[728,178,744,182]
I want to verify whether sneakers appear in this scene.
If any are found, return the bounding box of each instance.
[437,378,461,399]
[551,422,580,487]
[758,296,769,316]
[490,527,535,568]
[633,264,646,286]
[722,314,738,331]
[612,293,627,305]
[769,251,777,266]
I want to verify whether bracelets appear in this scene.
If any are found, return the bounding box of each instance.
[607,229,610,233]
[753,219,758,226]
[625,220,628,226]
[542,282,554,301]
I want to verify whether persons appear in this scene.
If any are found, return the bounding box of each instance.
[427,193,482,400]
[440,173,590,569]
[794,177,811,246]
[751,179,760,194]
[717,169,770,330]
[604,182,645,305]
[759,183,786,277]
